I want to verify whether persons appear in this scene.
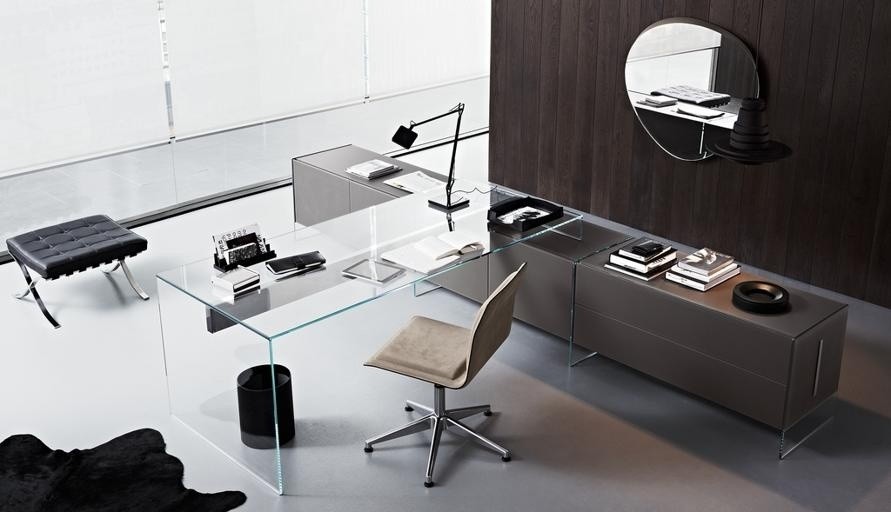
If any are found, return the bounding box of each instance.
[0,427,248,512]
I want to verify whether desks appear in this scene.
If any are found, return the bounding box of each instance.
[155,178,583,496]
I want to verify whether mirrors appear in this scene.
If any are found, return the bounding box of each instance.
[625,18,761,163]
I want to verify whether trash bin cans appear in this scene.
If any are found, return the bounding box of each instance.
[237,364,295,449]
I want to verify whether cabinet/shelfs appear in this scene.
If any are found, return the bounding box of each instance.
[291,142,848,462]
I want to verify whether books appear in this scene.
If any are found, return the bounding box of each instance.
[636,95,677,108]
[212,265,261,305]
[379,231,485,275]
[265,250,326,276]
[603,235,744,293]
[345,158,403,181]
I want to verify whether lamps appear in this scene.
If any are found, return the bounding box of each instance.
[392,103,470,209]
[705,1,793,165]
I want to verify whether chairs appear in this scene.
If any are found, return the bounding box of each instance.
[363,263,529,487]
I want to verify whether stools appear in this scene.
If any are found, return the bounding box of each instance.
[6,215,150,328]
[651,86,732,105]
